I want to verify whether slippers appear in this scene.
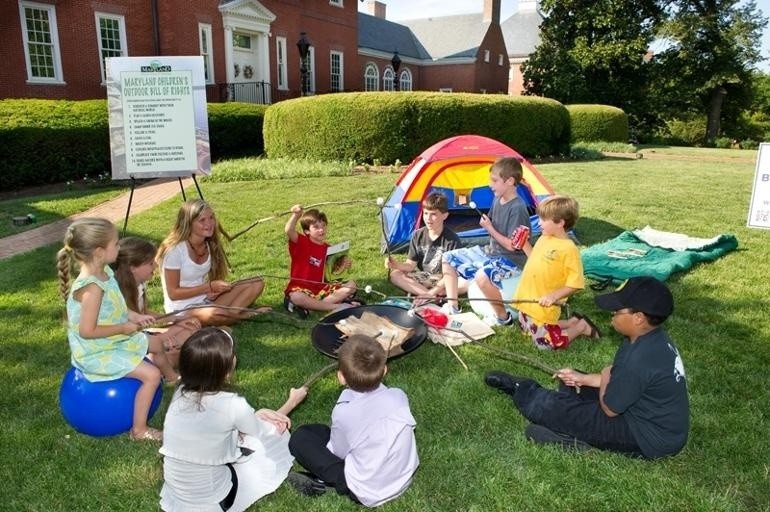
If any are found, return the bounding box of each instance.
[582,315,603,338]
[244,305,274,319]
[165,374,179,387]
[130,427,163,442]
[574,312,584,320]
[286,301,309,317]
[343,299,365,307]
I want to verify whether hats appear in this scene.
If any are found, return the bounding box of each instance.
[596,276,675,315]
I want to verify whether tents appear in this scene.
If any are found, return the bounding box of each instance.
[376,134,582,256]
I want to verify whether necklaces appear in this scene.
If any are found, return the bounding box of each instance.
[186,238,208,257]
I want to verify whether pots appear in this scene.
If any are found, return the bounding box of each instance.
[308,303,427,361]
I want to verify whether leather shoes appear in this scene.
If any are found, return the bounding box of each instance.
[485,370,533,393]
[526,424,591,449]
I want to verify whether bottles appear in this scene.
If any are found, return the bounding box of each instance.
[512,225,529,250]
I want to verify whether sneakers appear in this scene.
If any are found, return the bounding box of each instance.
[289,472,335,497]
[482,312,514,328]
[439,303,463,315]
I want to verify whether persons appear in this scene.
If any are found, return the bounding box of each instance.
[154,199,275,326]
[510,195,601,350]
[441,157,532,328]
[289,335,420,507]
[284,204,364,320]
[384,192,471,307]
[111,237,202,368]
[158,327,308,512]
[56,218,181,441]
[484,277,690,458]
